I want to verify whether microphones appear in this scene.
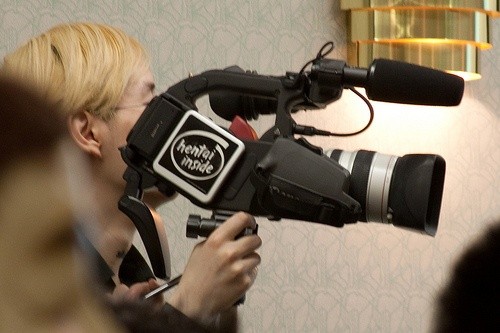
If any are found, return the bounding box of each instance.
[343,57,465,107]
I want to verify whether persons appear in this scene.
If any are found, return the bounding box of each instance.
[0,23,262,333]
